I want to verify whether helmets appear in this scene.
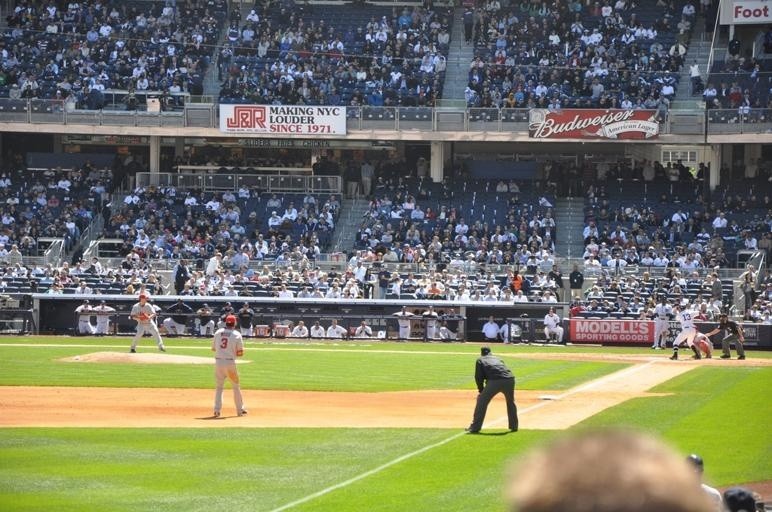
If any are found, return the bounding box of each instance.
[685,454,704,474]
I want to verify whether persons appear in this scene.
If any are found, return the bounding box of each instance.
[129,293,167,353]
[210,315,249,417]
[704,313,746,359]
[687,453,723,508]
[464,345,519,433]
[0,0,771,340]
[669,303,702,359]
[685,328,714,358]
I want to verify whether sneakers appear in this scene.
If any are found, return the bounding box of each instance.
[669,355,677,360]
[693,355,701,359]
[720,353,730,358]
[737,355,745,360]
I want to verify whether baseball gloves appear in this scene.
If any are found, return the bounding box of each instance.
[141,312,148,321]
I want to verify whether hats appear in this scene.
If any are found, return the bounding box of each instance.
[226,314,236,324]
[723,486,757,511]
[481,346,490,354]
